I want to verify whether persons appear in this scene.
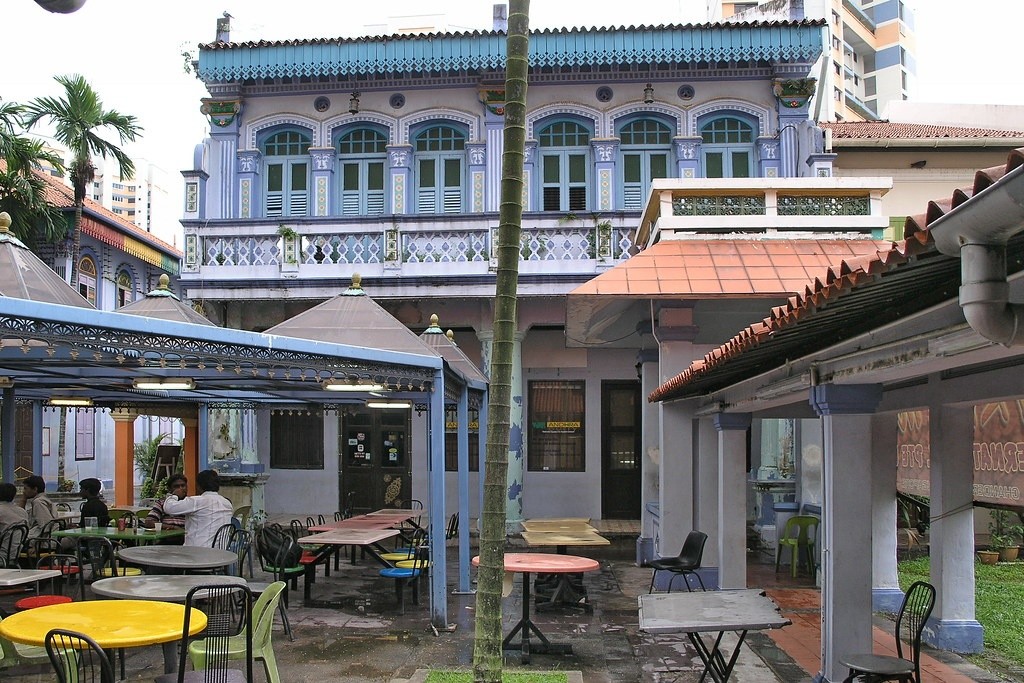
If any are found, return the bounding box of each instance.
[143,470,235,574]
[0,477,110,566]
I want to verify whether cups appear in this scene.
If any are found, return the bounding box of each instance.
[92,517,98,529]
[155,523,162,532]
[84,518,92,530]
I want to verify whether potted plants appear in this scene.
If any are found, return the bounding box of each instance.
[988,509,1024,561]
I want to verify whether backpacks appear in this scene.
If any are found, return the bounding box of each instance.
[258,526,303,568]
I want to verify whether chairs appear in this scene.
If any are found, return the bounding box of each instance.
[648,530,708,594]
[0,499,461,683]
[838,581,936,683]
[776,515,821,578]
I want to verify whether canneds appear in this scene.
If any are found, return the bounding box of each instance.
[118,518,125,531]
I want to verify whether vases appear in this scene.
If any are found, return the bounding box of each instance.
[978,550,999,565]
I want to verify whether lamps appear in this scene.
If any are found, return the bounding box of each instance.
[366,398,412,409]
[349,92,360,115]
[49,396,94,405]
[133,377,197,390]
[323,380,387,392]
[644,82,654,105]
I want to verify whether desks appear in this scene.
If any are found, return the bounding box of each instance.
[1,511,623,683]
[638,588,791,683]
